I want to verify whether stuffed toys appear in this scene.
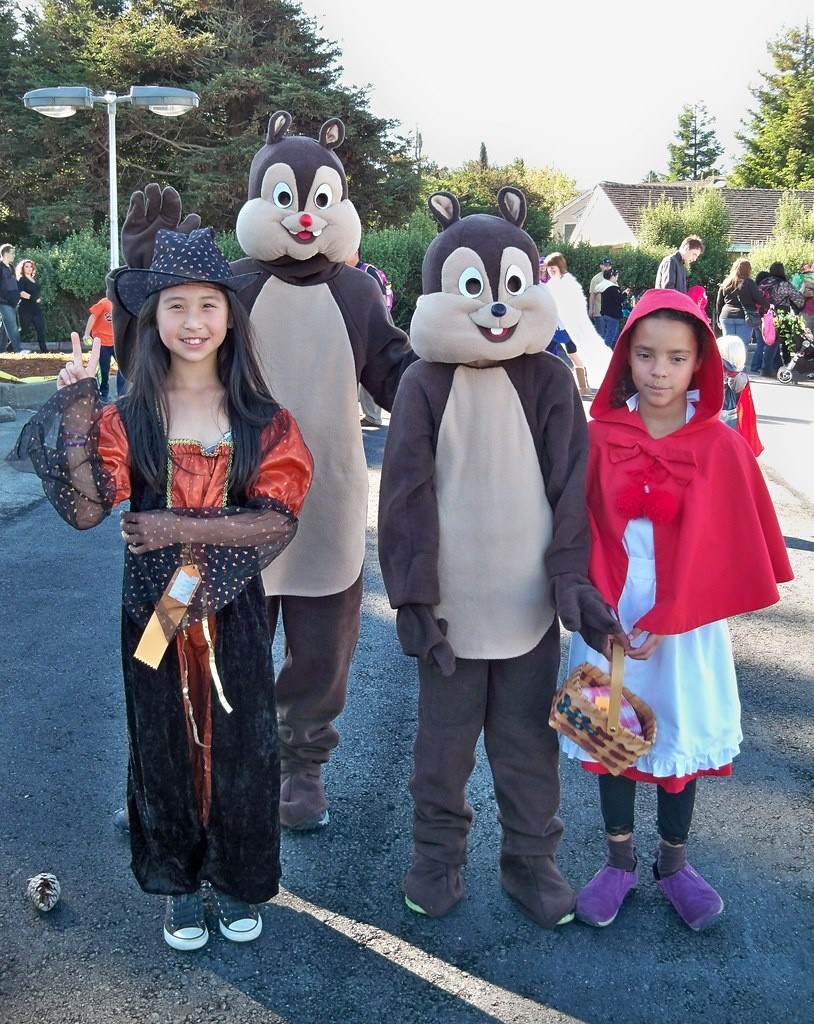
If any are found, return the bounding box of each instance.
[716,335,764,458]
[112,111,418,835]
[378,185,624,926]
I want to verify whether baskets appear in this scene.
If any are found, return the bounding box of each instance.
[548,638,658,777]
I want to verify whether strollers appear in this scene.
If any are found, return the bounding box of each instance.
[775,307,814,384]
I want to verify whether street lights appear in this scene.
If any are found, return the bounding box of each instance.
[21,83,201,273]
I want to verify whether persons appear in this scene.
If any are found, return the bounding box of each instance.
[578,286,794,931]
[6,260,51,354]
[717,259,814,377]
[589,257,630,348]
[83,297,127,404]
[0,244,31,354]
[8,226,315,950]
[655,237,703,293]
[344,244,386,428]
[542,251,592,396]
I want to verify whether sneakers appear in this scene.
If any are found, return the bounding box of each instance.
[163,889,207,951]
[208,882,262,942]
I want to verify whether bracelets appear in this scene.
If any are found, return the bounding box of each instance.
[61,431,93,445]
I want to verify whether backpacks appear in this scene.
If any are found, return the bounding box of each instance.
[754,278,788,312]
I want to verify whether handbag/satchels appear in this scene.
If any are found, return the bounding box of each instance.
[761,308,776,345]
[743,306,761,327]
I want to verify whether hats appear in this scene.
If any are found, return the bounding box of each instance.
[603,258,611,265]
[113,226,262,320]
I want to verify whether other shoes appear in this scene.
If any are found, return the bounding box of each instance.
[114,809,131,832]
[651,848,725,933]
[575,845,639,927]
[404,896,426,915]
[558,907,575,925]
[308,809,329,829]
[759,370,775,376]
